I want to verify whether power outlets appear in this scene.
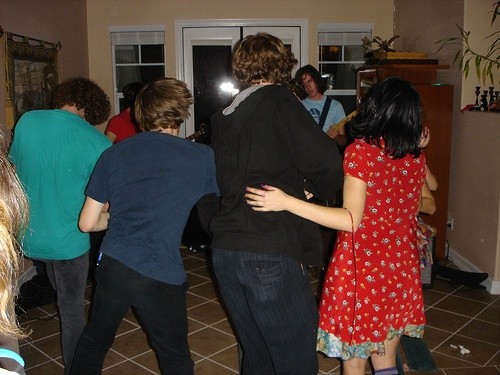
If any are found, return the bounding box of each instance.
[447,216,454,231]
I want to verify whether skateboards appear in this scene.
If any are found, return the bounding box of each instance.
[401,335,436,372]
[432,263,489,286]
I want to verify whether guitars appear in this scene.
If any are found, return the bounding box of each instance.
[185,123,208,142]
[325,101,366,137]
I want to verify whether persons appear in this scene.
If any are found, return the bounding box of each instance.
[77,77,218,375]
[8,76,111,375]
[246,76,440,375]
[100,81,151,145]
[285,64,347,139]
[209,31,342,375]
[0,129,31,375]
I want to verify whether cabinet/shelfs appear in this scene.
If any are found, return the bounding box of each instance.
[357,60,455,261]
